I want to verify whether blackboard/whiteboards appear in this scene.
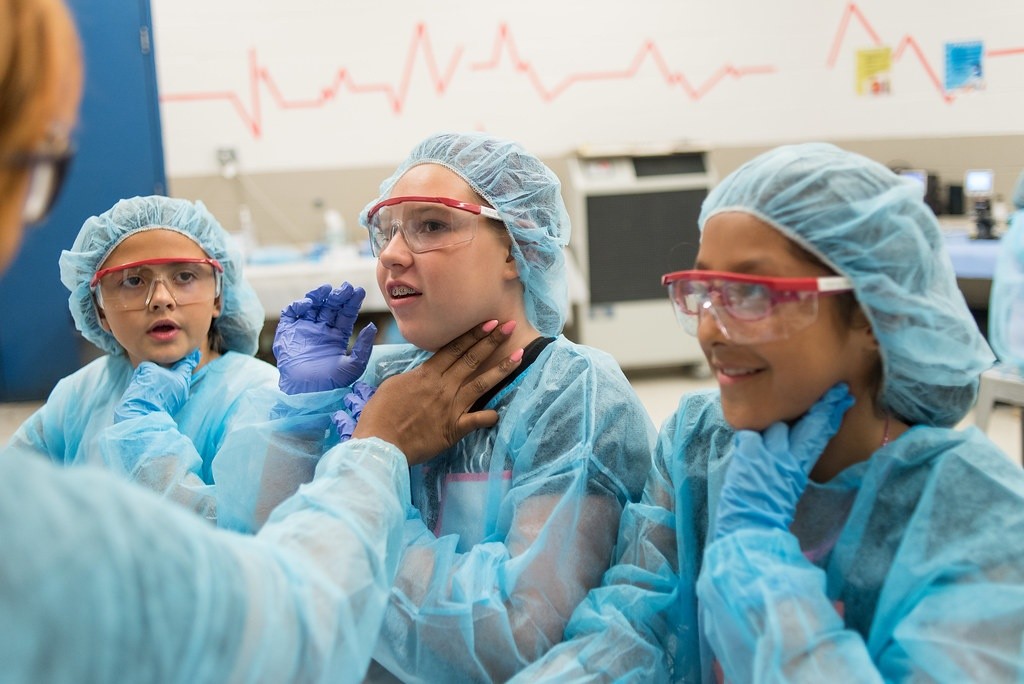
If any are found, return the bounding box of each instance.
[571,174,720,378]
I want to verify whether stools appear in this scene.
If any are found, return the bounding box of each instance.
[973,365,1024,435]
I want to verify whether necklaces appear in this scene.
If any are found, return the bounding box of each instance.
[879,407,891,447]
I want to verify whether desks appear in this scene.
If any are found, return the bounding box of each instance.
[941,228,1007,280]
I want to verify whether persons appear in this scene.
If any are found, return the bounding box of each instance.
[0,0,525,683]
[9,133,658,683]
[505,143,1024,684]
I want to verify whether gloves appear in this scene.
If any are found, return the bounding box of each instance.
[714,382,857,541]
[113,348,203,424]
[272,281,378,396]
[329,381,378,443]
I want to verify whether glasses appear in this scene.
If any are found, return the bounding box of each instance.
[367,196,535,259]
[661,270,852,346]
[90,257,224,312]
[0,150,71,224]
